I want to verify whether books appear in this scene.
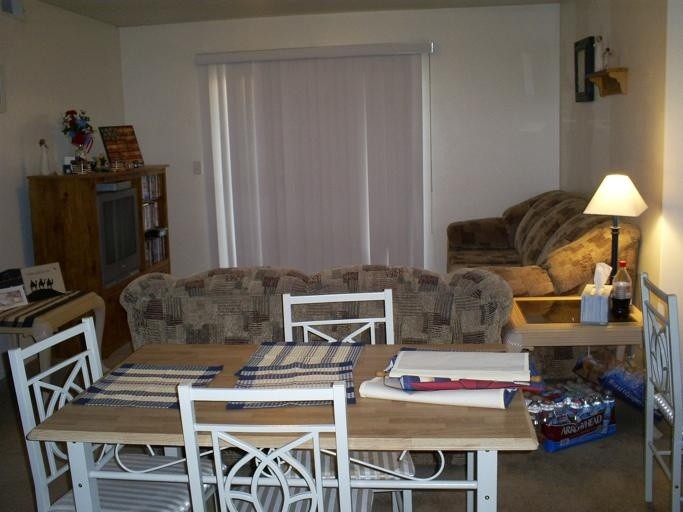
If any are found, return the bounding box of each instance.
[388,349,528,381]
[141,174,167,267]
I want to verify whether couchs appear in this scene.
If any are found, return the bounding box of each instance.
[447,189,642,379]
[120,267,511,352]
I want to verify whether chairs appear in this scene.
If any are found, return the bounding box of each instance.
[6,317,222,512]
[278,288,417,511]
[637,272,682,512]
[0,264,107,413]
[176,381,381,512]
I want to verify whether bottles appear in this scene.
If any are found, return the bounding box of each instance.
[74,143,88,175]
[526,378,617,454]
[610,261,633,319]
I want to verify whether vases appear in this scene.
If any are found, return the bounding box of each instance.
[74,144,88,171]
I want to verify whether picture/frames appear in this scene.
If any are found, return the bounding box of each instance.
[574,35,595,103]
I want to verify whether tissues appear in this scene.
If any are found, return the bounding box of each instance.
[580,262,613,327]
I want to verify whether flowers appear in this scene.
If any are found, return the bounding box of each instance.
[61,109,93,147]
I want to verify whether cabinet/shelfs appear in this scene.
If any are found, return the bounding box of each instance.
[25,162,173,363]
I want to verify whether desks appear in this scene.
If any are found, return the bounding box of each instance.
[26,343,541,512]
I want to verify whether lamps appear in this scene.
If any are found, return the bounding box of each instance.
[584,174,648,301]
